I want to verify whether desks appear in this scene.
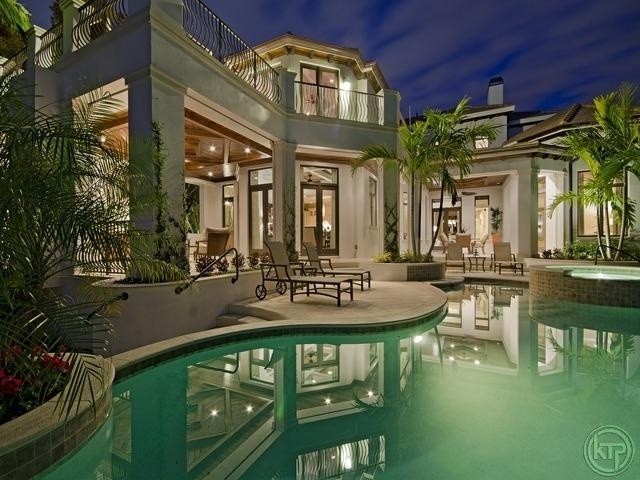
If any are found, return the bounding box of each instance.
[468,284,486,294]
[468,256,486,272]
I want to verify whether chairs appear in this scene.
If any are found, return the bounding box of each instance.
[255,239,354,308]
[490,241,524,276]
[195,227,231,263]
[490,287,523,307]
[439,231,489,255]
[444,244,466,273]
[298,241,371,292]
